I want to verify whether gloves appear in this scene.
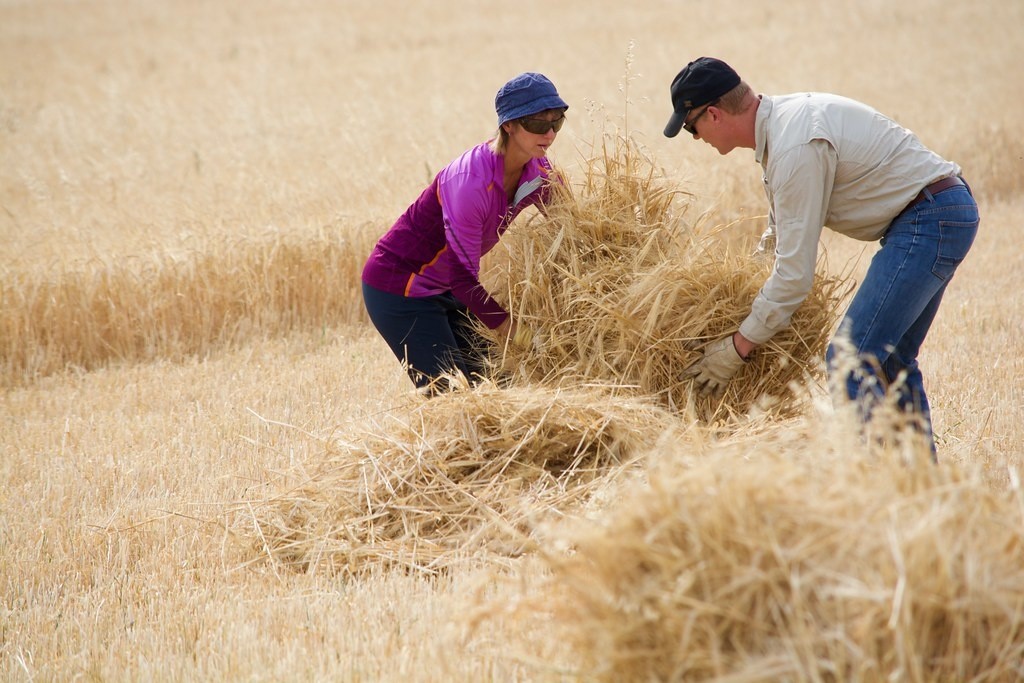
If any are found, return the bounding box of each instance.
[513,323,534,352]
[677,335,750,400]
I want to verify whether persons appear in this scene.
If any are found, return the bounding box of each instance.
[663,59,980,468]
[361,74,578,402]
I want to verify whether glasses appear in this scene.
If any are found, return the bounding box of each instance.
[683,98,720,135]
[514,114,564,135]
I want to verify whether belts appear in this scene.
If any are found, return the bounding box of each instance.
[903,177,970,212]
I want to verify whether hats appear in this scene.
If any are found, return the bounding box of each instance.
[495,72,569,129]
[662,57,740,137]
